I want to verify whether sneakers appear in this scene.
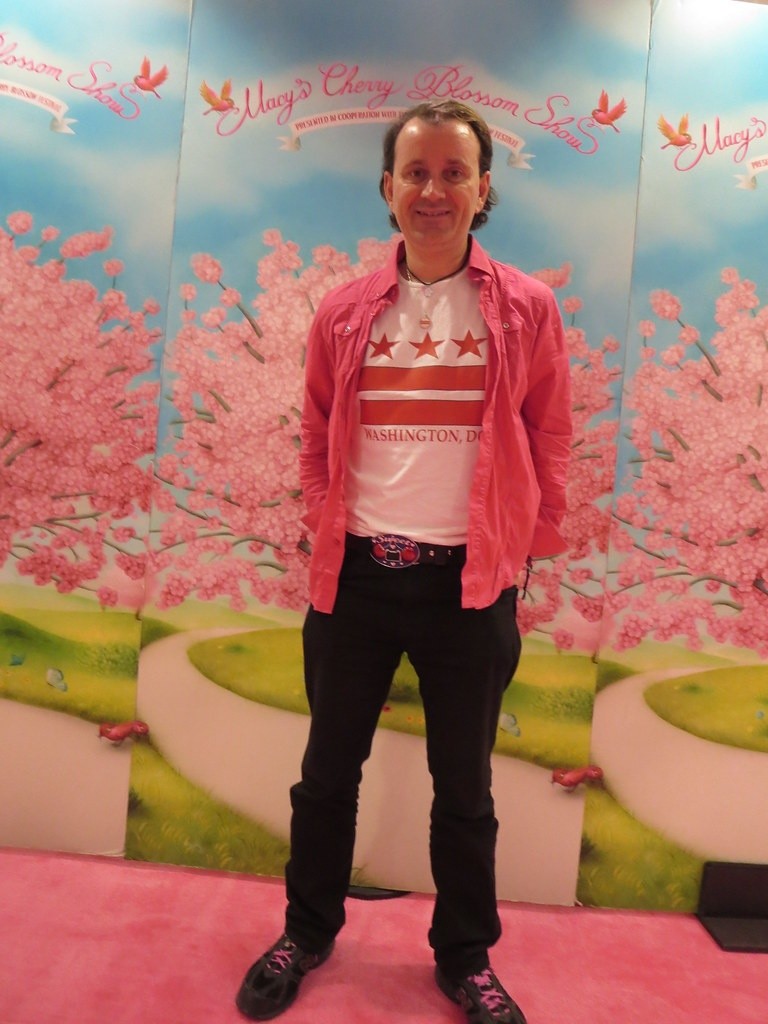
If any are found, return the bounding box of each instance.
[236,931,338,1020]
[434,957,527,1024]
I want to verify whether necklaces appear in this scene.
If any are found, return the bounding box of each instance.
[405,264,465,284]
[407,270,412,281]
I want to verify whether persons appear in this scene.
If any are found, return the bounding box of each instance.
[236,101,576,1024]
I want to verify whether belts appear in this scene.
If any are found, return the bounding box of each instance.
[348,532,468,569]
